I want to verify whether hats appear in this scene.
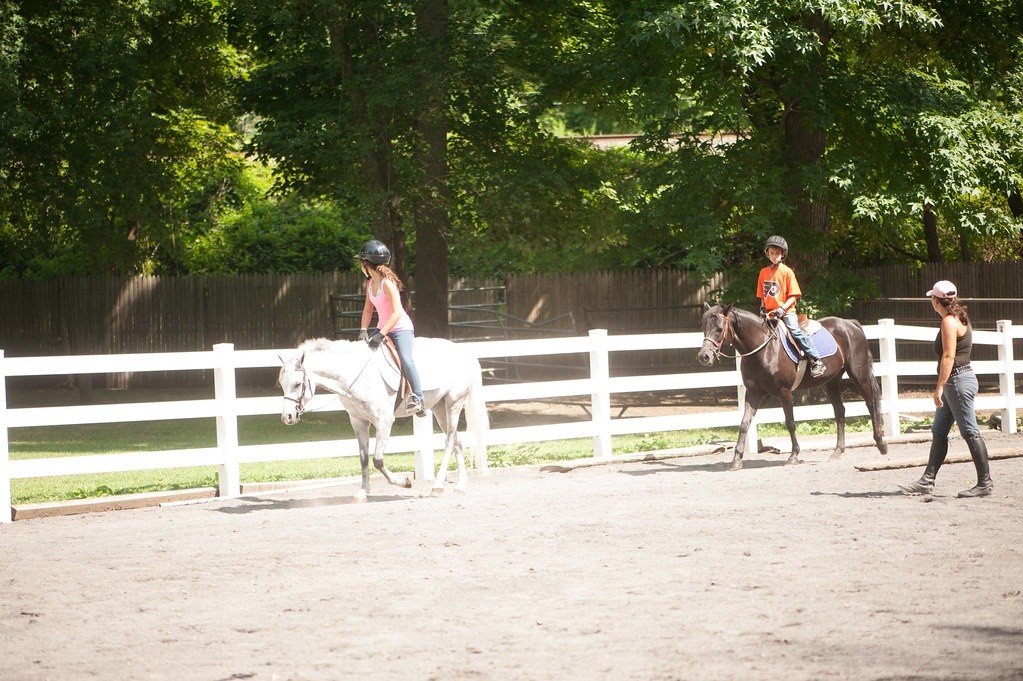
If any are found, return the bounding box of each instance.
[926,280,957,298]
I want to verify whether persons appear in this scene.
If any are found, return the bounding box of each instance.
[897,280,994,499]
[359,239,426,418]
[756,235,827,378]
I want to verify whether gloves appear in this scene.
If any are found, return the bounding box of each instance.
[775,307,785,318]
[357,330,368,340]
[368,332,385,350]
[759,307,766,317]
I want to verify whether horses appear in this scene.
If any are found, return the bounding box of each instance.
[278,337,490,503]
[696,303,889,471]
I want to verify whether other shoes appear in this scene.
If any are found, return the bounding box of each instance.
[408,398,426,417]
[958,483,994,498]
[897,481,933,495]
[811,358,826,378]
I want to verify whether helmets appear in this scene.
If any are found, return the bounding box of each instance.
[764,236,788,261]
[353,240,391,265]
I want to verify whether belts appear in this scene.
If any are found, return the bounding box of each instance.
[949,365,972,378]
